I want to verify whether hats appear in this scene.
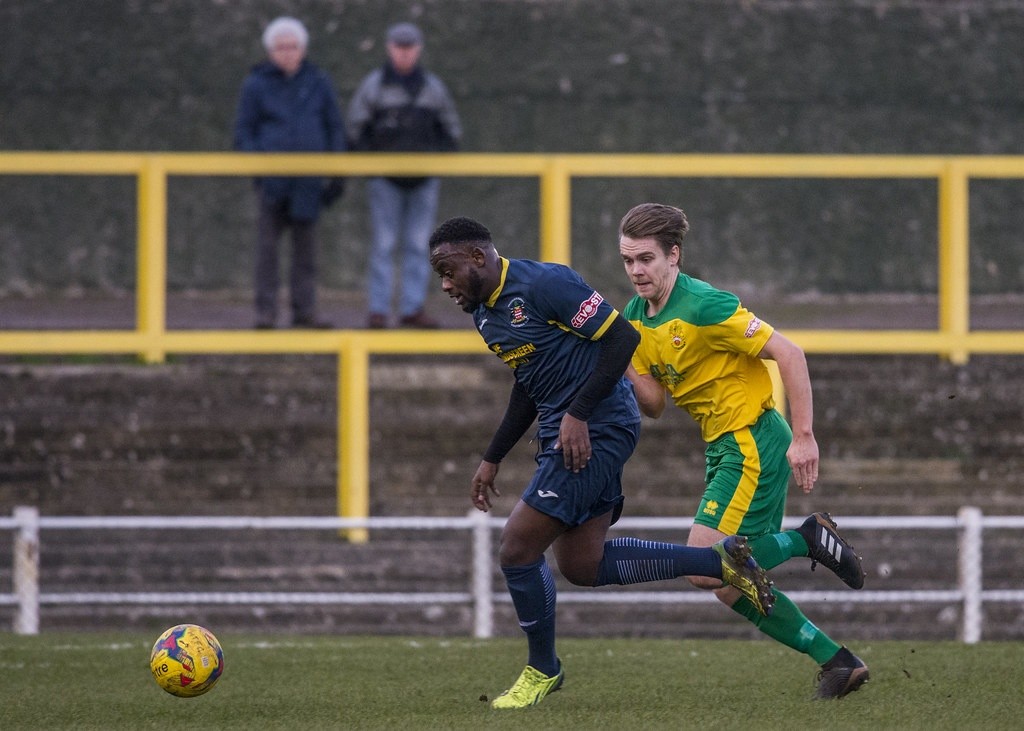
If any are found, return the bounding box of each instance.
[386,22,424,46]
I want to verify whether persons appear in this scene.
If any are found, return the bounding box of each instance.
[429,217,777,708]
[345,19,465,330]
[622,203,871,702]
[231,16,351,329]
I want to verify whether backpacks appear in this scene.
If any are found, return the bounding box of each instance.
[368,106,443,188]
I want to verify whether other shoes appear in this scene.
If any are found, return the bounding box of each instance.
[256,314,275,329]
[401,317,438,329]
[370,316,384,328]
[293,313,335,329]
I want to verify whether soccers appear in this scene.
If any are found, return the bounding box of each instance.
[147,622,227,699]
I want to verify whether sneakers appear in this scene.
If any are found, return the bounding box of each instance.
[802,512,867,589]
[809,642,869,701]
[712,536,777,616]
[491,657,564,709]
